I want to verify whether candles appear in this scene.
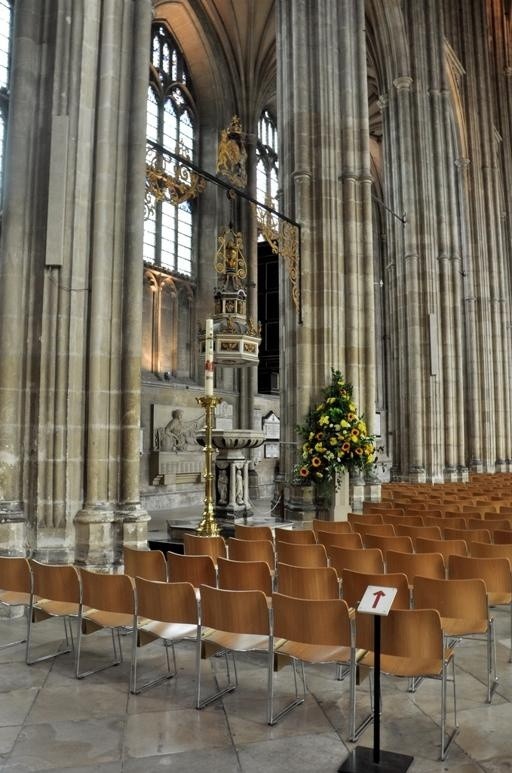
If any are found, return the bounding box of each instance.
[204,318,215,397]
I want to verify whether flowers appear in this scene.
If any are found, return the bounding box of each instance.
[295,367,387,485]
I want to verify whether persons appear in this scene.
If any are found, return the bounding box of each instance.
[165,409,209,451]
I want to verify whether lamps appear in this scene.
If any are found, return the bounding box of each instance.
[198,191,262,368]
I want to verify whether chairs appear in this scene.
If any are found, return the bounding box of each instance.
[1,470,509,764]
